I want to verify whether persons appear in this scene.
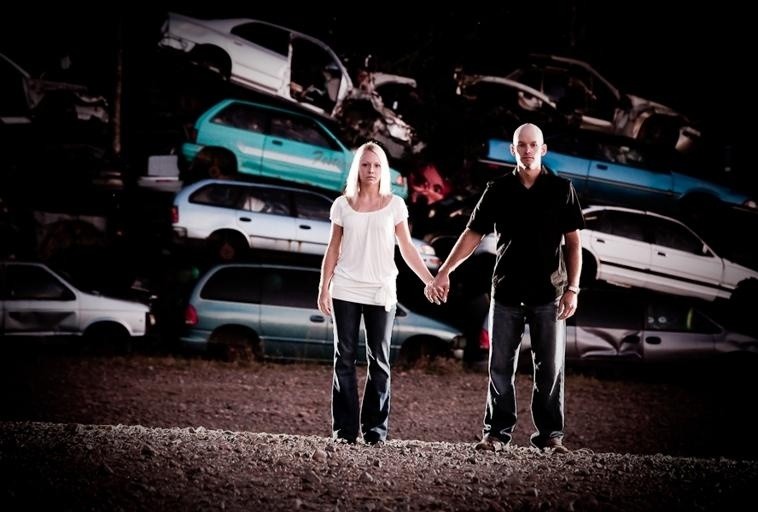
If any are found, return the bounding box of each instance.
[316,140,444,444]
[424,122,587,454]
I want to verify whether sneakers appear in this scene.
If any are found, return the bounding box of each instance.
[474,438,510,452]
[541,435,570,454]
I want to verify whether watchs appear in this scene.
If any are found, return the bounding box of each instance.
[567,285,580,294]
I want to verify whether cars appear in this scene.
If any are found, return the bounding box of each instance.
[1,261,161,360]
